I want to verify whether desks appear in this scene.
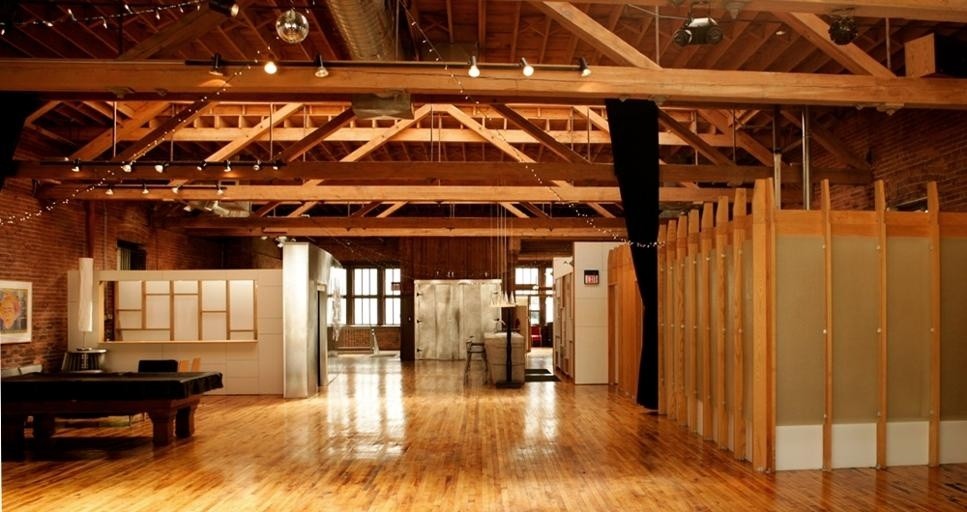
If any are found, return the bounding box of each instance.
[0,371,223,452]
[65,349,106,370]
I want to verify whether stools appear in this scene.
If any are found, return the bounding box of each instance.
[464,340,486,377]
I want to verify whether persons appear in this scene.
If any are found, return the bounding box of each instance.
[0,292,23,329]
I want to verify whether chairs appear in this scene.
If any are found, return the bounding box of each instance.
[136,356,200,373]
[530,326,542,346]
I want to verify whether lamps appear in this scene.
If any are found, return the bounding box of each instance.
[670,0,725,46]
[68,157,281,196]
[205,51,328,80]
[76,257,94,352]
[466,54,590,79]
[208,1,241,19]
[826,10,859,46]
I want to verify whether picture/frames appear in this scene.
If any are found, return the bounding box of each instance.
[0,279,34,346]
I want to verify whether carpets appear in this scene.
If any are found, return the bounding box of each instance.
[524,368,560,382]
